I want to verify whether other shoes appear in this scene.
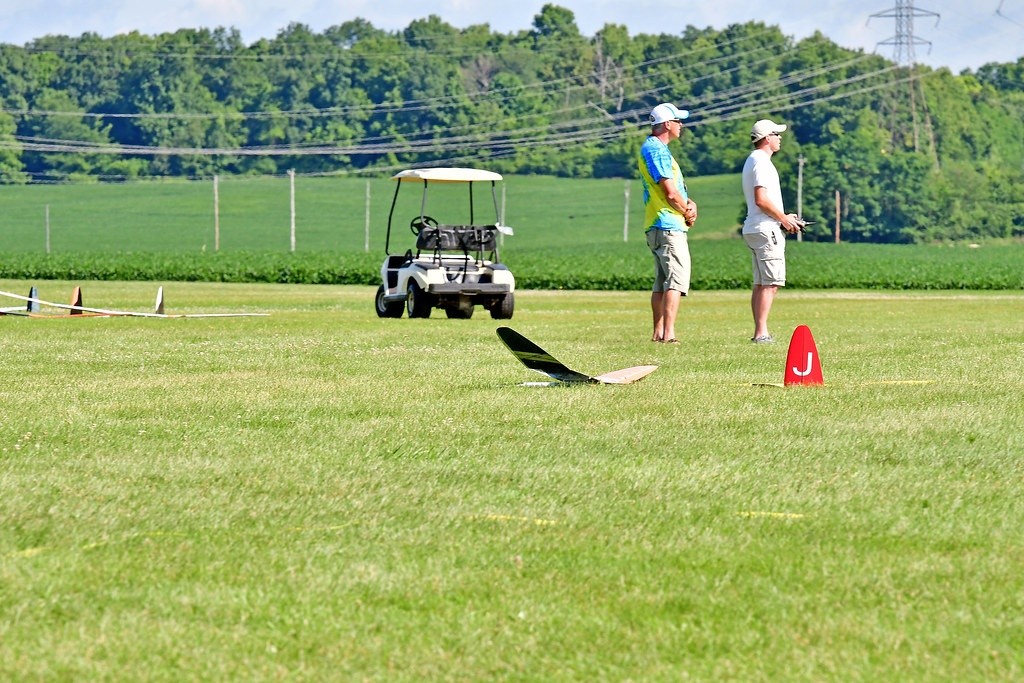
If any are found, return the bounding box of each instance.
[652,336,680,345]
[753,334,773,342]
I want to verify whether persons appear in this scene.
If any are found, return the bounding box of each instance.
[742,119,801,342]
[639,103,698,344]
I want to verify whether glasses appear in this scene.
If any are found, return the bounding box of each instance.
[669,119,680,123]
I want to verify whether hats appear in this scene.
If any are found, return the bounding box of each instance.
[751,120,787,143]
[649,103,689,125]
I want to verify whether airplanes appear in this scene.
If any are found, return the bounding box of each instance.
[713,324,933,387]
[497,327,659,386]
[0,284,273,319]
